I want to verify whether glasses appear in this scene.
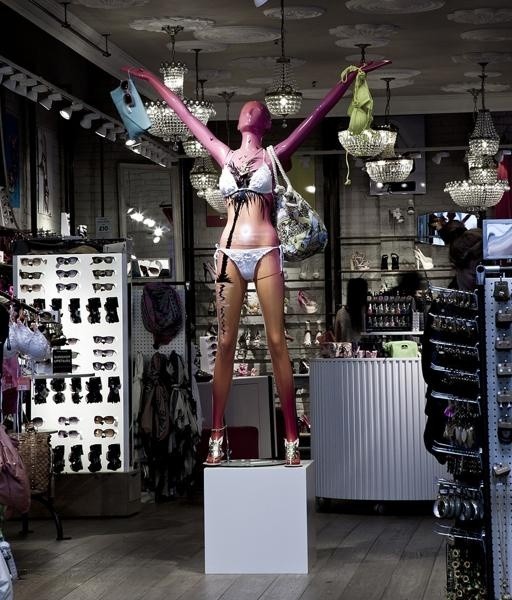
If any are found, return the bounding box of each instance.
[33,377,121,404]
[19,256,116,293]
[53,444,121,473]
[121,80,136,108]
[33,416,119,441]
[22,298,119,324]
[67,337,118,370]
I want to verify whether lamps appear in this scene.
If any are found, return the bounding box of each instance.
[260,0,302,130]
[137,20,217,145]
[180,47,222,160]
[0,59,169,170]
[123,201,176,280]
[188,77,217,194]
[199,92,244,223]
[341,43,512,215]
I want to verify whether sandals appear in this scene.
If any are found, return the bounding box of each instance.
[207,435,223,461]
[285,438,301,465]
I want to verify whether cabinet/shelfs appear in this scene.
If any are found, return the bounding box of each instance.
[192,247,333,450]
[203,463,315,576]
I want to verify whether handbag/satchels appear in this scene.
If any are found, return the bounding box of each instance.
[111,84,152,139]
[1,429,53,518]
[272,189,329,261]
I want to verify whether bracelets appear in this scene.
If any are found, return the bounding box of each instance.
[432,495,485,521]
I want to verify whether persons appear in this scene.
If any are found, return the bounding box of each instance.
[329,278,368,348]
[420,220,493,464]
[121,60,393,465]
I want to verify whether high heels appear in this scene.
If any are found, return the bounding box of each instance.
[202,259,323,375]
